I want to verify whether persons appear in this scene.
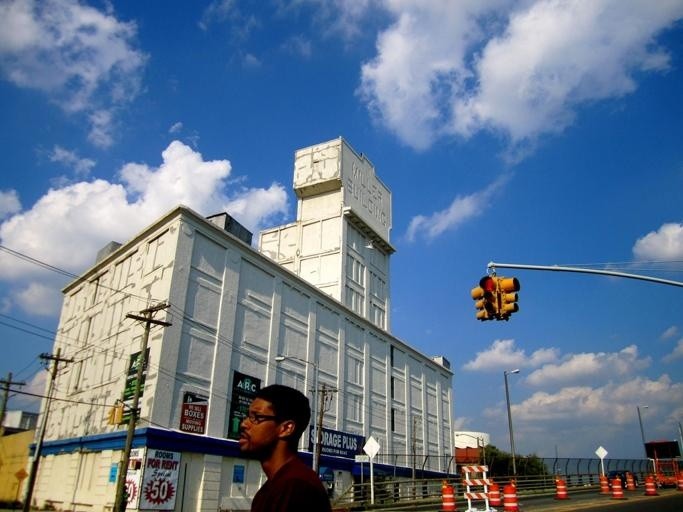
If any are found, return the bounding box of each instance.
[238,384,331,511]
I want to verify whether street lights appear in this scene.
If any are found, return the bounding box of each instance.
[274,355,318,473]
[503,369,520,475]
[637,405,649,445]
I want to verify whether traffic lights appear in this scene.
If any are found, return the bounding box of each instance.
[107,409,114,424]
[500,275,521,316]
[481,275,498,317]
[471,286,484,323]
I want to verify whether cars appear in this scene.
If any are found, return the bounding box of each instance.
[606,470,638,488]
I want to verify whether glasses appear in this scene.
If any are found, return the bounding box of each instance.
[247,410,273,423]
